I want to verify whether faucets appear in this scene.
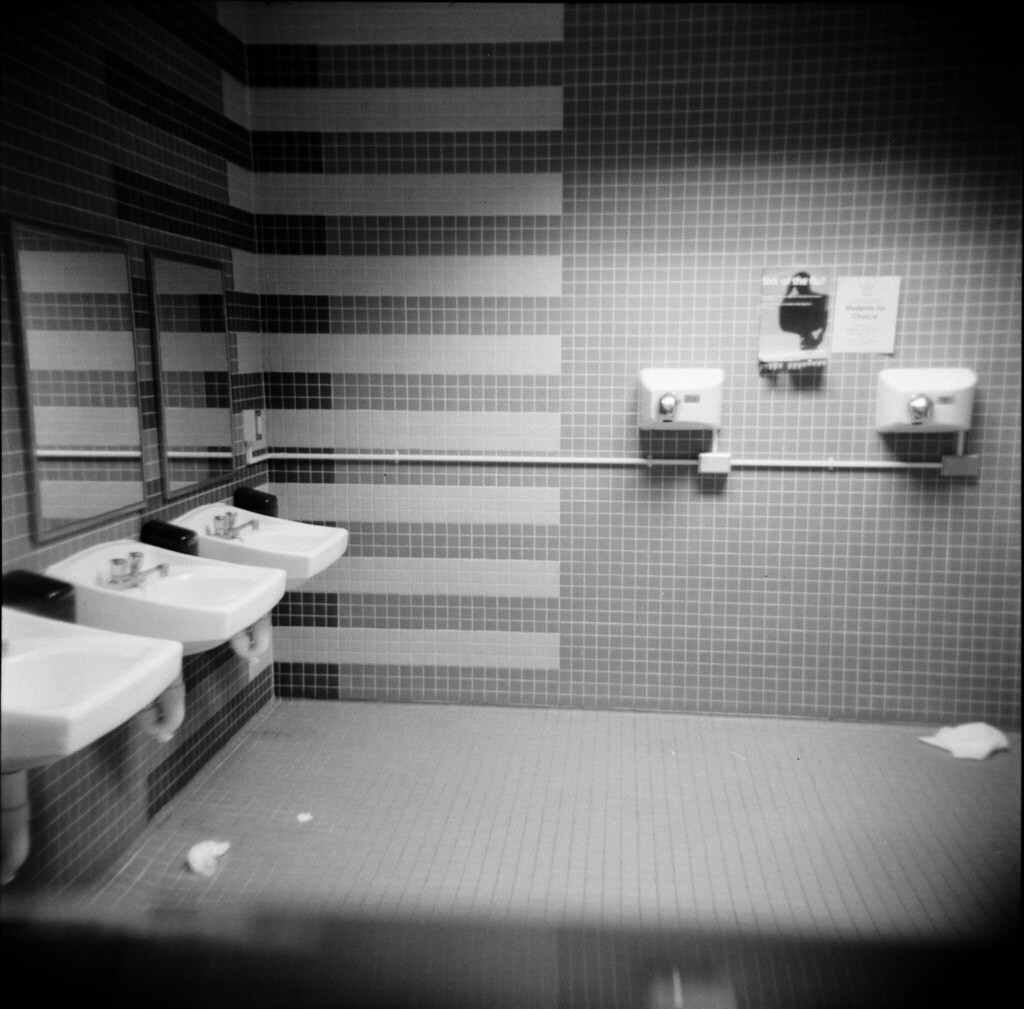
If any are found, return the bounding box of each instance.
[229,519,261,537]
[131,563,171,584]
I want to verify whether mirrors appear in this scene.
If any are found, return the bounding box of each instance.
[2,213,149,548]
[144,245,238,505]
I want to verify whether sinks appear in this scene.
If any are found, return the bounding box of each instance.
[3,608,183,772]
[35,537,288,657]
[160,500,349,591]
